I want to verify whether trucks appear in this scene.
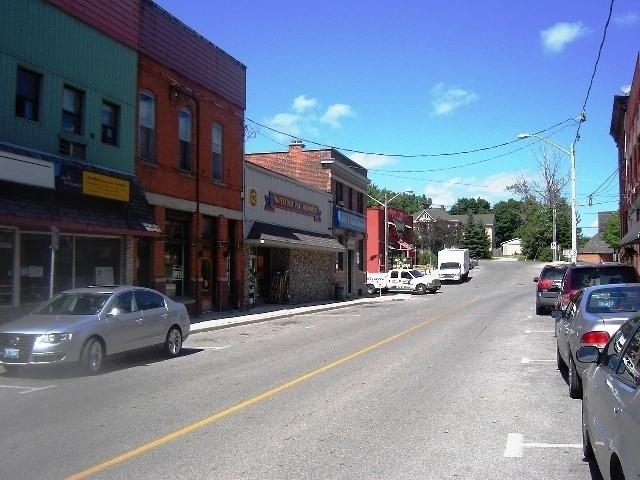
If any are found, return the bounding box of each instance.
[435,247,471,286]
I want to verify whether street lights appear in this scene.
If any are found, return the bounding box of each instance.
[383,190,415,272]
[513,130,579,264]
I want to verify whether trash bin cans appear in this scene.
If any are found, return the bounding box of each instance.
[335,287,345,301]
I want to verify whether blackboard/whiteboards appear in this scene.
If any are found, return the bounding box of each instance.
[96,267,114,285]
[28,265,43,277]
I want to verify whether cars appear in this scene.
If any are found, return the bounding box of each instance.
[0,281,192,378]
[532,260,640,480]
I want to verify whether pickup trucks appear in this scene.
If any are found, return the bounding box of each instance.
[364,268,443,296]
